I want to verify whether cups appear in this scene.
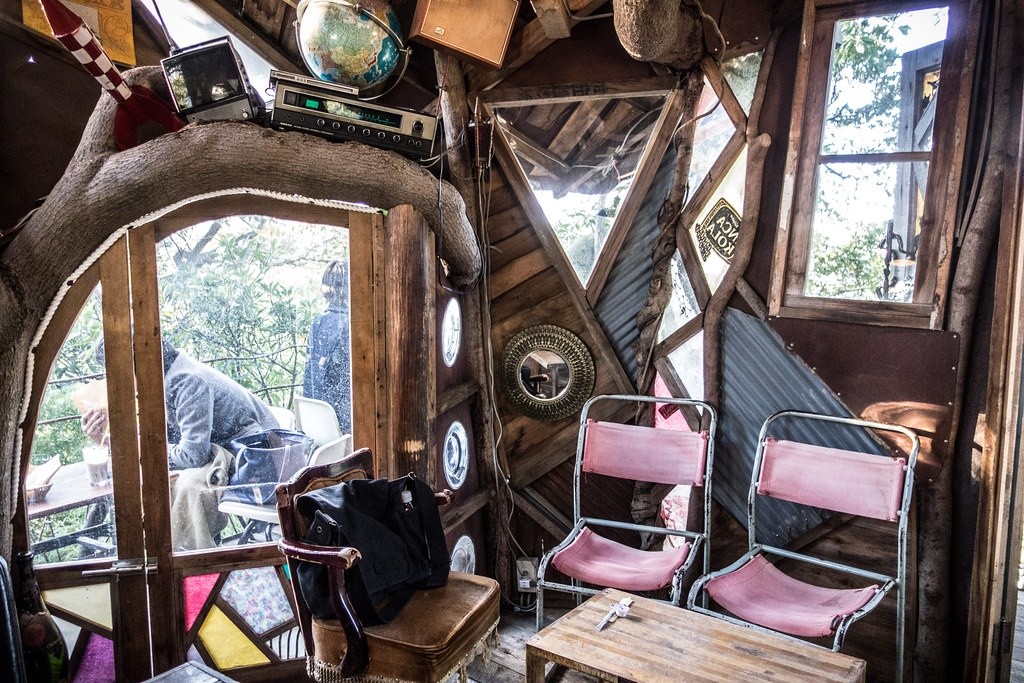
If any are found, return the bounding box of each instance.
[83,443,109,486]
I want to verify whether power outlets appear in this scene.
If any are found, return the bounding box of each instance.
[517,557,538,593]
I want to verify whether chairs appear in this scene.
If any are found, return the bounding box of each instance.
[294,397,343,443]
[276,447,500,683]
[688,409,918,683]
[537,395,719,629]
[219,433,353,544]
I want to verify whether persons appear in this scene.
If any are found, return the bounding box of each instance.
[303,259,351,438]
[81,332,282,562]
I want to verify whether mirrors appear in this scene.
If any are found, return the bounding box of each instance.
[517,349,570,400]
[484,89,668,288]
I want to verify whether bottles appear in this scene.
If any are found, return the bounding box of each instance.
[14,550,70,683]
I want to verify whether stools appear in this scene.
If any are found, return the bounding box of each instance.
[142,660,237,683]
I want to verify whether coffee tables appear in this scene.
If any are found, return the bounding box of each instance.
[526,587,867,683]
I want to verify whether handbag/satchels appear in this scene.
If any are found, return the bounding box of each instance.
[300,473,449,626]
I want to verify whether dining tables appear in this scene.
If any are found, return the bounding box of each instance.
[28,460,180,566]
